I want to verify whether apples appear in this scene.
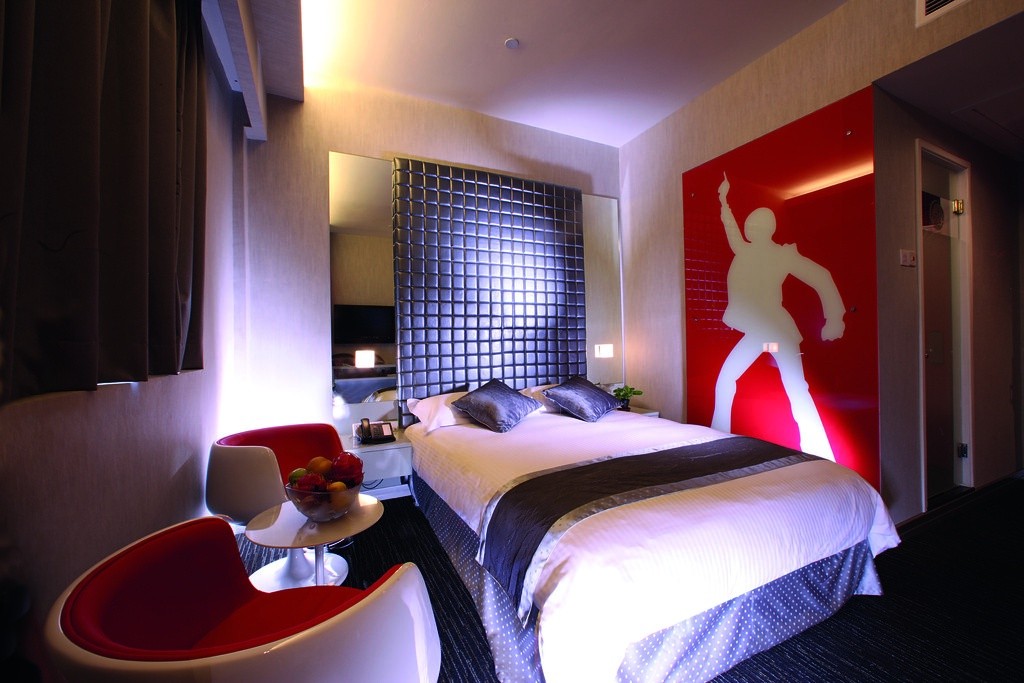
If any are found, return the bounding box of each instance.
[288,468,307,487]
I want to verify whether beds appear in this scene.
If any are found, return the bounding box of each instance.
[404,409,902,682]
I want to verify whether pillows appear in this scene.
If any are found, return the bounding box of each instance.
[518,383,560,412]
[452,377,543,433]
[406,391,472,436]
[541,376,625,422]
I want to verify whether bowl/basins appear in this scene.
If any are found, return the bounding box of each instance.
[285,482,360,523]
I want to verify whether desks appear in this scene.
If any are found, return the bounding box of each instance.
[245,493,384,585]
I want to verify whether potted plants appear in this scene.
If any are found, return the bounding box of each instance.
[614,386,642,412]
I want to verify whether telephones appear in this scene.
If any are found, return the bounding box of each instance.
[355,418,396,446]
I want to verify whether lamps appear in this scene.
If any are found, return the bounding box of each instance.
[354,348,375,368]
[594,344,614,359]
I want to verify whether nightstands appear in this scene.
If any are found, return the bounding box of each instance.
[339,432,418,506]
[618,405,659,417]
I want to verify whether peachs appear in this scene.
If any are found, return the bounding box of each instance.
[327,481,351,505]
[305,457,333,475]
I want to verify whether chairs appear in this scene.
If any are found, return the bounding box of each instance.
[43,515,441,683]
[203,423,349,594]
